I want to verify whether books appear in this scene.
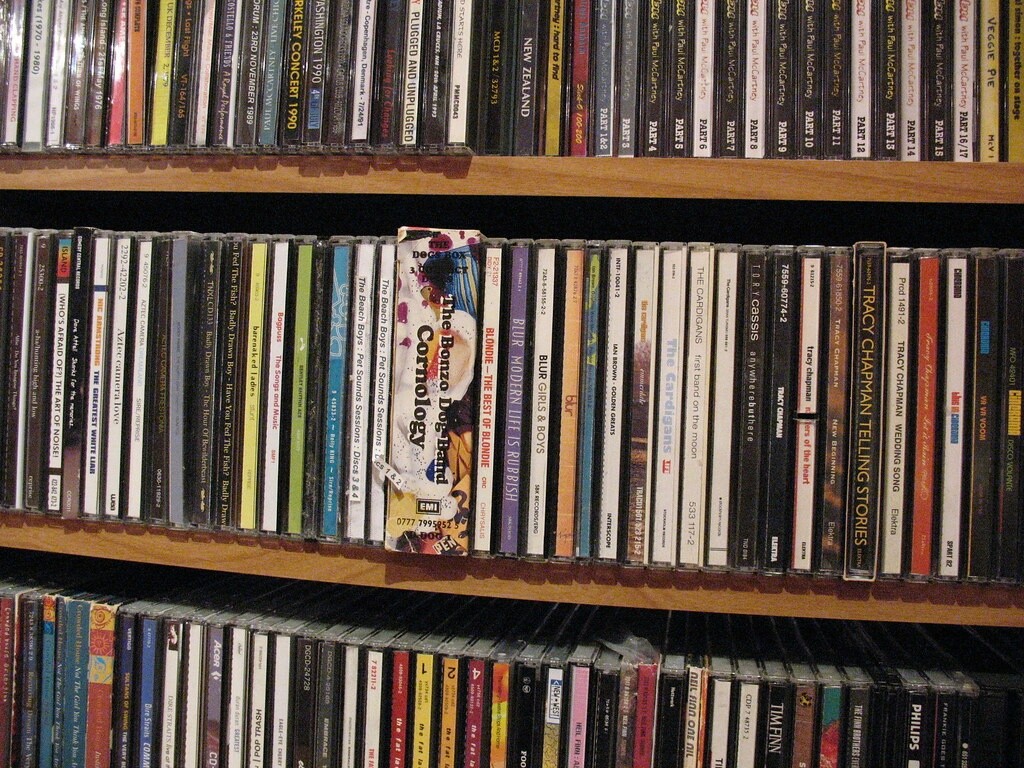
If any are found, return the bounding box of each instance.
[0,225,1024,582]
[0,1,1023,160]
[0,582,1024,768]
